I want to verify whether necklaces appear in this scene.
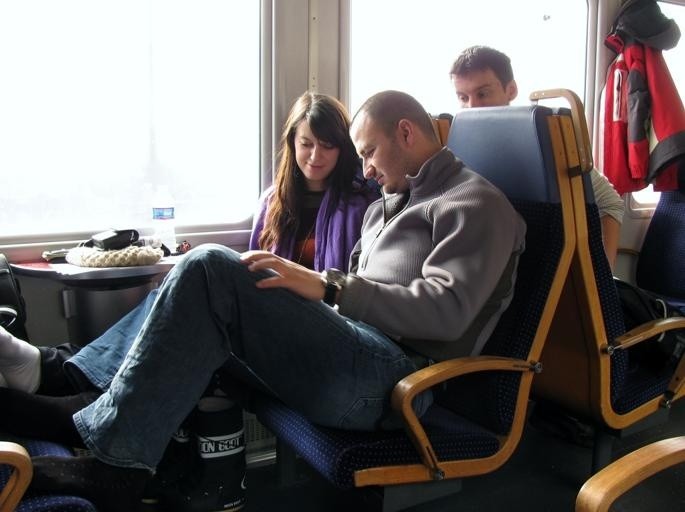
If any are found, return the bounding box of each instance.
[297,220,316,265]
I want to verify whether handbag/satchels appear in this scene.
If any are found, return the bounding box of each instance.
[616,279,684,373]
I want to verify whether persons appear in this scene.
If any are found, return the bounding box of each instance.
[0,91,379,398]
[0,90,527,503]
[448,45,626,280]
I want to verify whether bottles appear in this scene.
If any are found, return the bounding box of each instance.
[152,186,177,252]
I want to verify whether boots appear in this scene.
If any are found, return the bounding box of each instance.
[160,394,248,511]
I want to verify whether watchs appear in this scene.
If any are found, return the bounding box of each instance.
[322,268,345,307]
